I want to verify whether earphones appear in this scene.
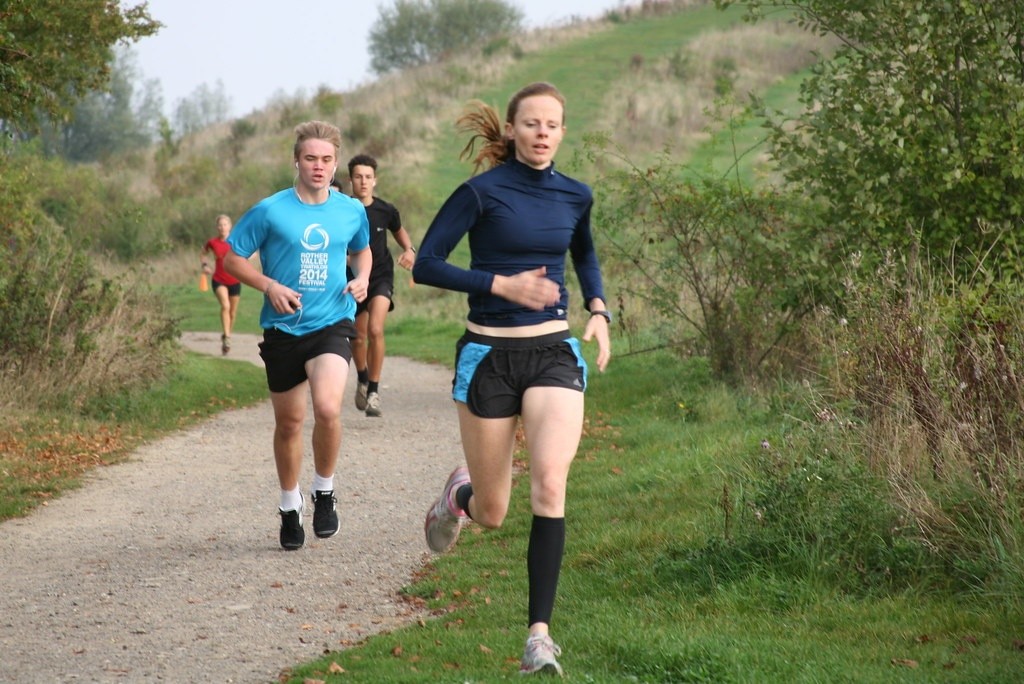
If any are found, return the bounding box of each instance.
[295,162,298,169]
[333,167,336,173]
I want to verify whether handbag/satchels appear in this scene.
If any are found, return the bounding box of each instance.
[199,273,207,291]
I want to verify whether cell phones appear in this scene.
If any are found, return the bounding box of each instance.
[288,297,301,310]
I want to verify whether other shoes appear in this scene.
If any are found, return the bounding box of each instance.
[221,334,231,355]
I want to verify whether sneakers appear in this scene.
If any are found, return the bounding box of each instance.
[278,491,305,550]
[424,466,473,554]
[311,489,340,539]
[365,391,381,417]
[355,379,368,411]
[520,633,563,679]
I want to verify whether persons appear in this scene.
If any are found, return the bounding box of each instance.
[199,215,258,353]
[412,82,610,677]
[327,155,417,416]
[224,120,373,549]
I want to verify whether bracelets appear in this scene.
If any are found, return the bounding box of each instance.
[589,311,611,323]
[202,263,207,267]
[411,247,416,253]
[265,280,277,294]
